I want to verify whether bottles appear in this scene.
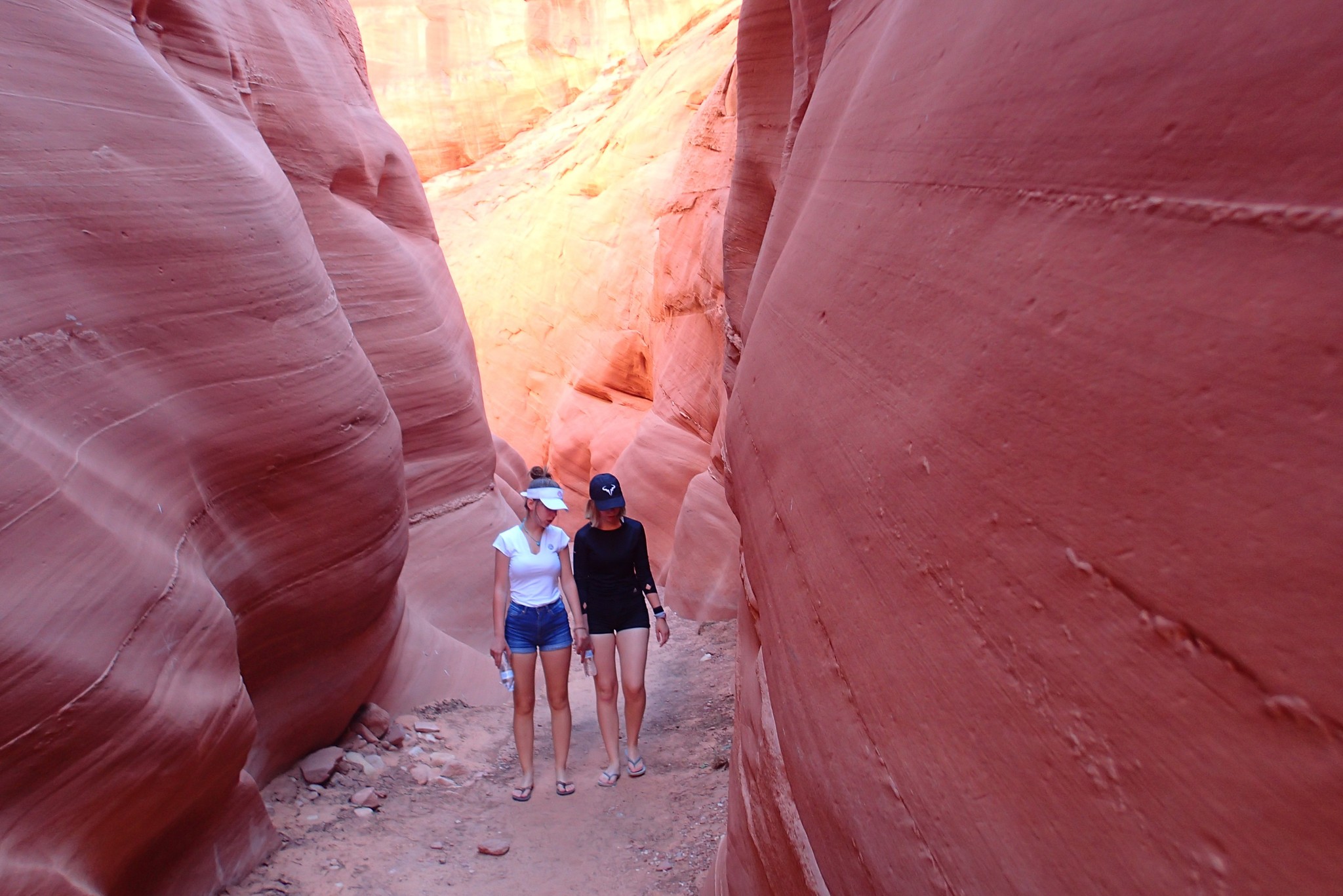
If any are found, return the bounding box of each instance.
[583,648,597,677]
[499,651,514,692]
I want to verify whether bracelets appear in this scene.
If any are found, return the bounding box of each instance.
[573,627,586,633]
[653,606,664,614]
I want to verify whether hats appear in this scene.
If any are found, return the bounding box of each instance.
[589,473,625,511]
[520,487,569,512]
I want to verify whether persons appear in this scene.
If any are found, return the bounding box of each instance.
[490,467,590,801]
[574,473,670,787]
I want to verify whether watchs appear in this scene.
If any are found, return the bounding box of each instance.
[655,613,665,620]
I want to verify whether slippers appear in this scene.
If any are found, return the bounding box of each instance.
[511,784,534,800]
[598,772,620,786]
[624,749,646,776]
[556,781,575,795]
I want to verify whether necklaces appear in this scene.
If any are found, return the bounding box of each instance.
[523,519,542,546]
